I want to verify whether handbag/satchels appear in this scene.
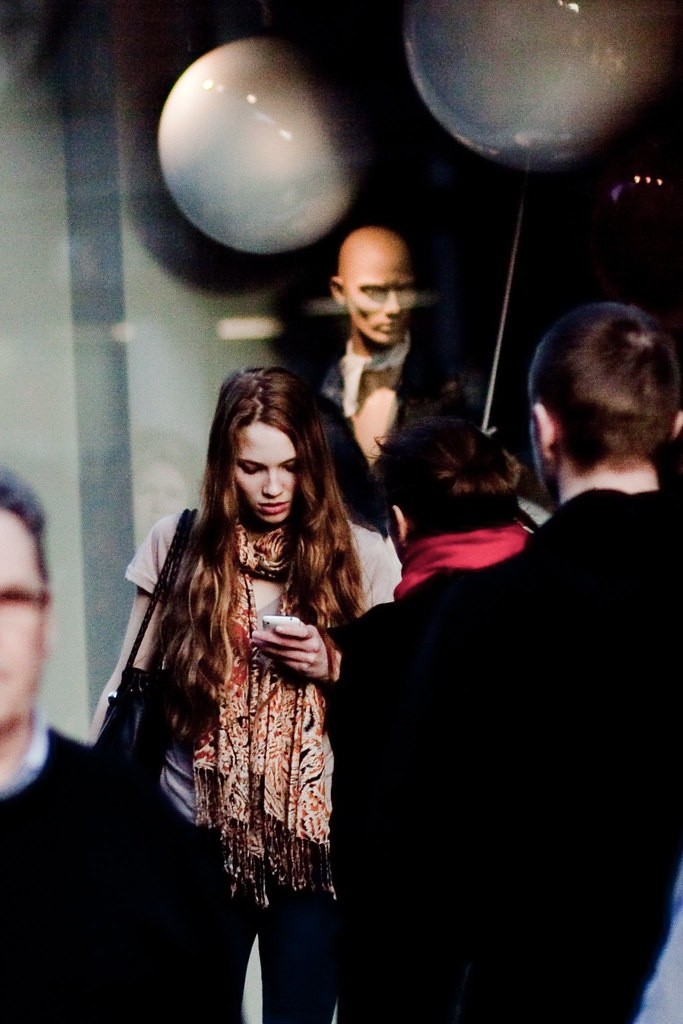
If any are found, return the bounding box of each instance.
[98,509,197,762]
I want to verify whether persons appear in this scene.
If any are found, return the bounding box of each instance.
[0,367,403,1024]
[324,304,682,1024]
[323,226,485,466]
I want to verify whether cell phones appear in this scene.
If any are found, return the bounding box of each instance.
[262,615,300,633]
